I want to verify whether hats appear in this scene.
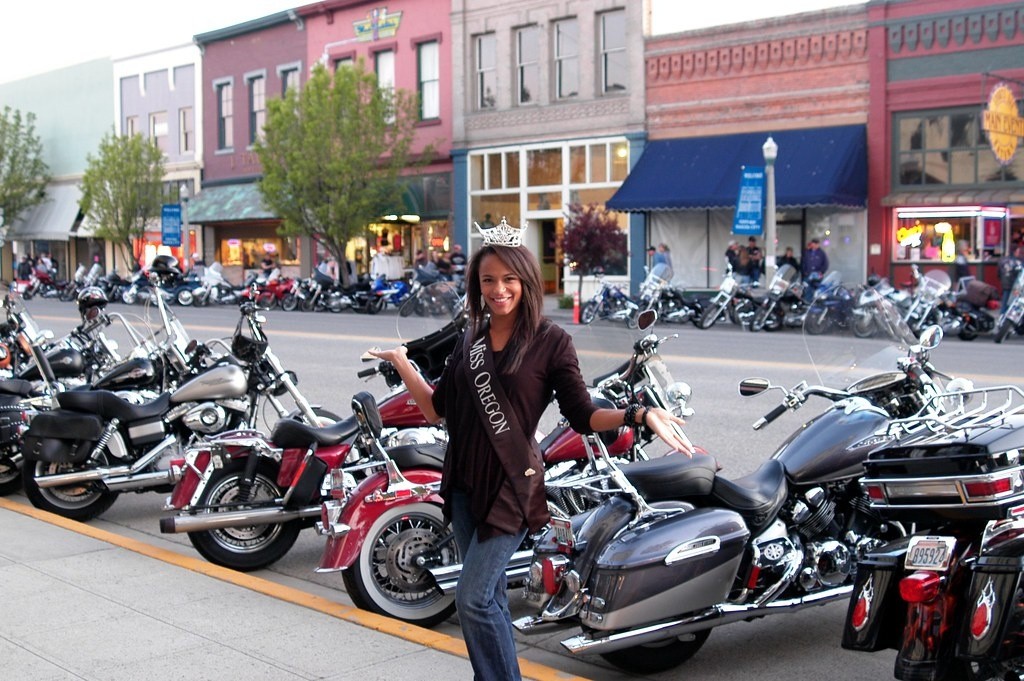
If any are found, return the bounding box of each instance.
[748,236,756,242]
[646,246,656,251]
[728,240,737,247]
[811,238,820,243]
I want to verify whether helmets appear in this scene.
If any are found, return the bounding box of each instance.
[78,287,109,318]
[231,333,270,362]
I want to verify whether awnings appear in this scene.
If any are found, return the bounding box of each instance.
[79,179,127,237]
[605,124,869,211]
[6,184,87,240]
[184,183,294,223]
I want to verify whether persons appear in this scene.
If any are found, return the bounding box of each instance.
[414,245,466,289]
[192,253,205,267]
[261,252,275,276]
[133,260,140,272]
[725,237,763,283]
[779,247,799,282]
[17,252,59,279]
[801,239,828,286]
[369,216,693,681]
[645,243,674,282]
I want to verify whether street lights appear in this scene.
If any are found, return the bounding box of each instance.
[761,135,779,291]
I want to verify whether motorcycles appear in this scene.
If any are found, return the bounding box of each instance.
[160,280,473,575]
[749,263,818,333]
[626,262,727,332]
[991,258,1023,345]
[314,310,725,629]
[578,267,647,326]
[699,253,784,333]
[802,271,870,336]
[0,302,130,497]
[18,270,367,523]
[901,263,999,344]
[841,386,1024,681]
[510,277,974,675]
[0,259,467,319]
[849,266,936,340]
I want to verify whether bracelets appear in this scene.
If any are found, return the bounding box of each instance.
[624,404,643,428]
[642,406,651,426]
